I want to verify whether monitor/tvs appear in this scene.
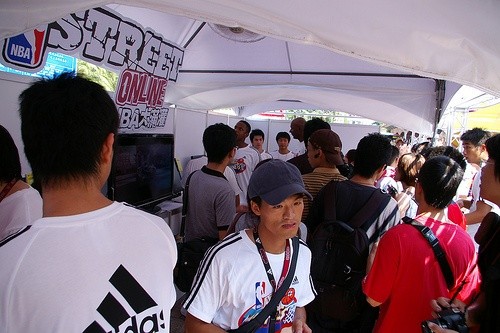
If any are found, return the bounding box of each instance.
[108,132,183,214]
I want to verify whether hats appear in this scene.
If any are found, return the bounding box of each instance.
[309,129,344,165]
[247,159,313,204]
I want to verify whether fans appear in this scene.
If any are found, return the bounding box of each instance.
[208,22,266,42]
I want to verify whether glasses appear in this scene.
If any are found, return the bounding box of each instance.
[481,155,495,164]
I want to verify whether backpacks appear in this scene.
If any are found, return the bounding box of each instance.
[306,181,388,321]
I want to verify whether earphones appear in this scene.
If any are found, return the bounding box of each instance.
[314,153,320,158]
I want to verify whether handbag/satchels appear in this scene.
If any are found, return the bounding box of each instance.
[173,235,220,292]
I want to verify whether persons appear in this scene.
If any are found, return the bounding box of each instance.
[183,117,500,333]
[0,126,42,234]
[0,71,178,333]
